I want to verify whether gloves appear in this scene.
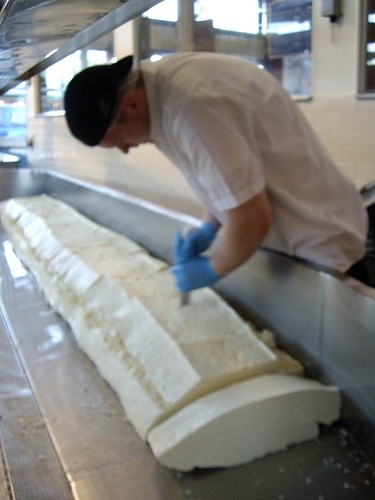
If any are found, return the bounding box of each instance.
[171,256,225,292]
[173,219,217,264]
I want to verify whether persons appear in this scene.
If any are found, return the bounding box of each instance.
[64,51,375,294]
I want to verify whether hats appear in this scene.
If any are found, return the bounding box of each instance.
[64,55,133,147]
